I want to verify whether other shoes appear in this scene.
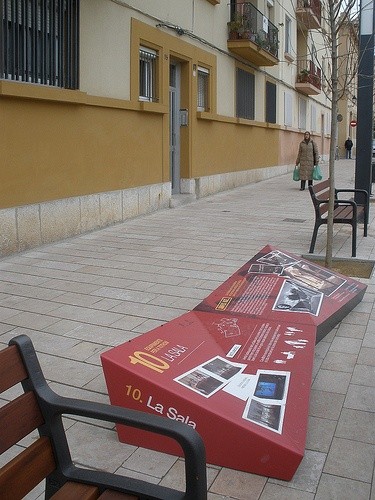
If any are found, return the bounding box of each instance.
[300,188,305,191]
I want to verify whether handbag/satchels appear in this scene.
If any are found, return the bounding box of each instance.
[312,164,322,180]
[293,165,299,181]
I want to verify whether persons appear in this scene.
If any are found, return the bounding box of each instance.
[295,131,319,191]
[344,137,353,159]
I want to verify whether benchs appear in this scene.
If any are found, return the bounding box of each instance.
[308,178,369,258]
[0,334,208,500]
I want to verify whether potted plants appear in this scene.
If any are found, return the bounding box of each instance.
[227,13,276,57]
[297,71,321,88]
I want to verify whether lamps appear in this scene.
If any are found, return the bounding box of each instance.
[348,96,356,109]
